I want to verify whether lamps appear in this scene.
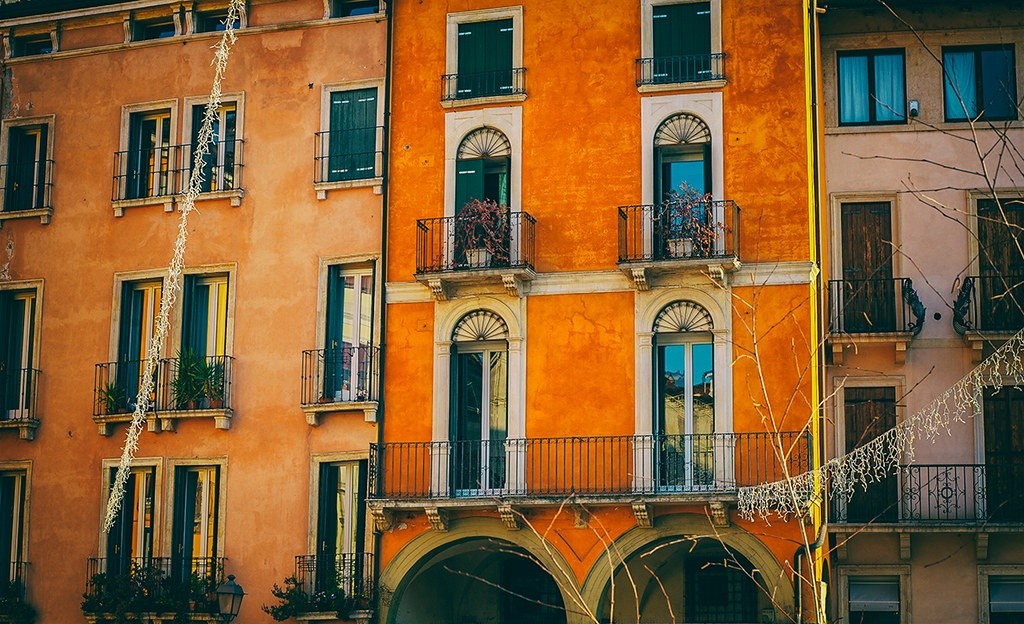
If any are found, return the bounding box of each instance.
[216,575,245,624]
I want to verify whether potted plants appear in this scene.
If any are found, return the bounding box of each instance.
[318,383,334,402]
[354,389,368,402]
[0,576,36,624]
[168,345,224,409]
[81,565,215,624]
[95,381,128,414]
[261,574,371,622]
[450,199,515,268]
[651,181,732,257]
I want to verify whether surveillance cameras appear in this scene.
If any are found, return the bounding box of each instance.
[909,99,919,116]
